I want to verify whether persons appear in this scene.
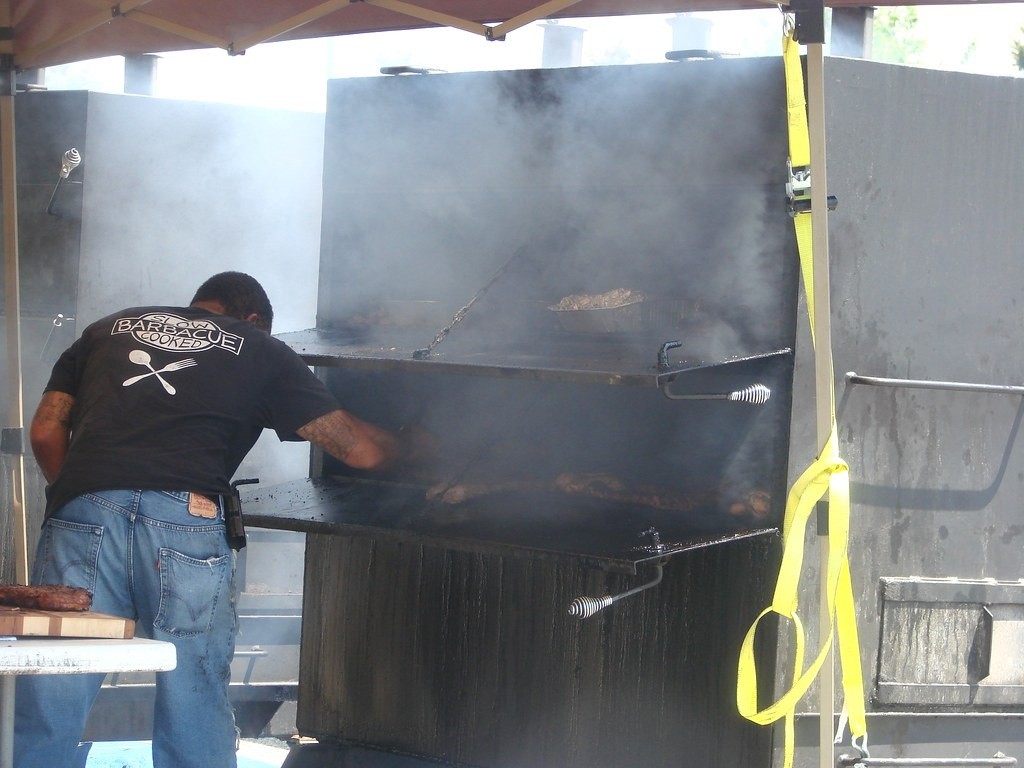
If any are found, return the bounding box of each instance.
[12,270,442,768]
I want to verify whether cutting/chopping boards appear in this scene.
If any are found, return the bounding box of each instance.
[0,604,136,640]
